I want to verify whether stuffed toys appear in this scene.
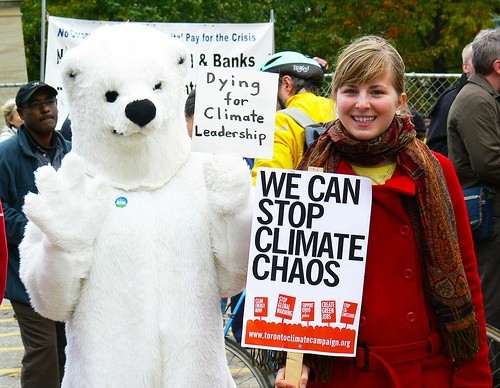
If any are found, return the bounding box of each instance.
[18,24,254,388]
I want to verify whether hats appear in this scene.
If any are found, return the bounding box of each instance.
[15,81,57,107]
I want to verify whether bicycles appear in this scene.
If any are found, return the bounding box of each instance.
[222,290,288,388]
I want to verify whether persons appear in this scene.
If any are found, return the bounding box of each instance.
[411,29,500,388]
[274,38,492,388]
[0,81,73,388]
[252,52,337,184]
[185,90,245,344]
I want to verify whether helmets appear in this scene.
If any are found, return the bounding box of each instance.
[260,51,324,88]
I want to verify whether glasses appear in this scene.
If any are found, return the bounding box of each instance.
[21,99,56,108]
